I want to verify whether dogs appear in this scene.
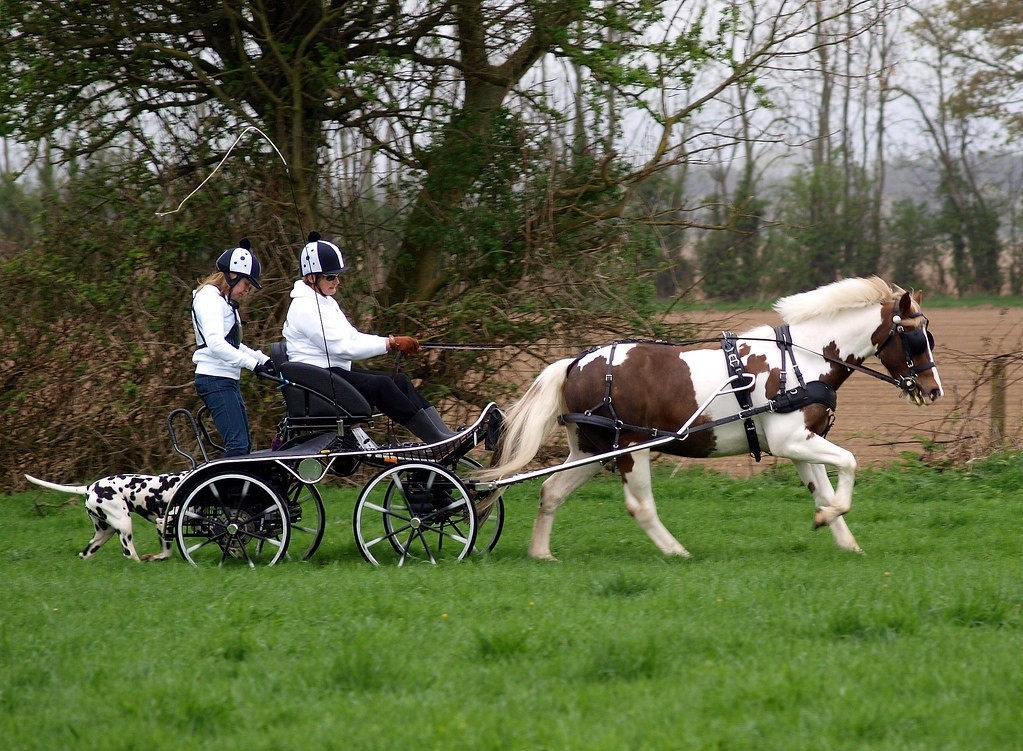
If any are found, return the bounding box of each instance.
[25,419,208,565]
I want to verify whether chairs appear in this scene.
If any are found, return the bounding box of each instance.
[270,337,384,422]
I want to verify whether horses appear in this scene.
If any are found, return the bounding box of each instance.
[462,274,946,564]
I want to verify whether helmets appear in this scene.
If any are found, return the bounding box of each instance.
[216,238,263,289]
[299,231,351,276]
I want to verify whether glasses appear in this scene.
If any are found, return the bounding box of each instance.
[320,273,339,281]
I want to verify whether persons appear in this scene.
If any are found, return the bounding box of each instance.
[282,239,465,453]
[191,247,273,454]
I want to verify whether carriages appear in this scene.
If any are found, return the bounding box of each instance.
[154,271,944,572]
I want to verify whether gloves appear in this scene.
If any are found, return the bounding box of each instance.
[388,335,419,356]
[263,359,277,378]
[255,361,269,379]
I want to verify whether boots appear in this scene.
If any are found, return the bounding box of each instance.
[403,405,468,459]
